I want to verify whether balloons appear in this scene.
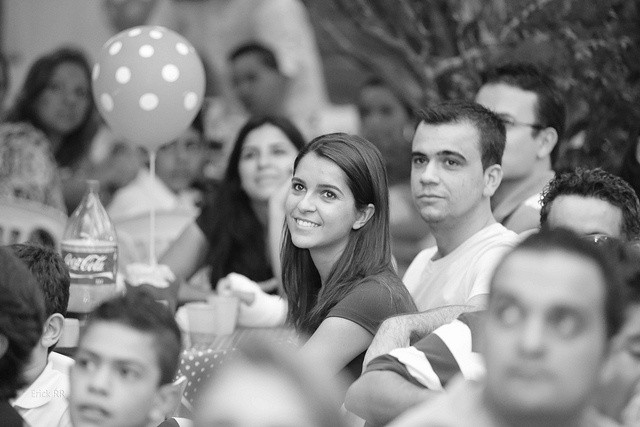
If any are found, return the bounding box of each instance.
[90,25,206,151]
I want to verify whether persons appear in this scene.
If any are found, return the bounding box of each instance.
[0,39,640,427]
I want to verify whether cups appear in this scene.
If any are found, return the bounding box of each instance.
[125,263,239,355]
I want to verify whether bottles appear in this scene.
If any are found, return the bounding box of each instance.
[55,178,120,326]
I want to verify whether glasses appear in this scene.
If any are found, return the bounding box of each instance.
[497,112,544,131]
[584,234,611,248]
